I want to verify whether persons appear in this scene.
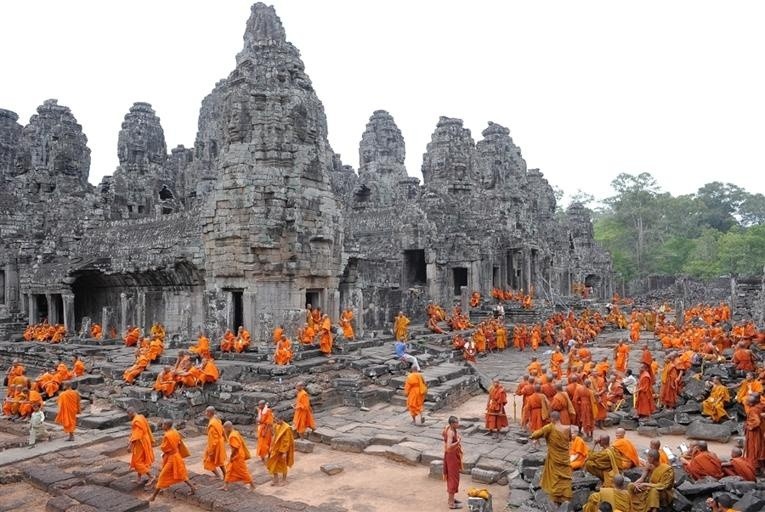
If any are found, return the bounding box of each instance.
[394,284,764,512]
[220,326,252,354]
[291,382,317,442]
[127,406,295,501]
[124,322,221,398]
[271,323,293,365]
[23,318,116,344]
[3,354,85,449]
[296,304,357,358]
